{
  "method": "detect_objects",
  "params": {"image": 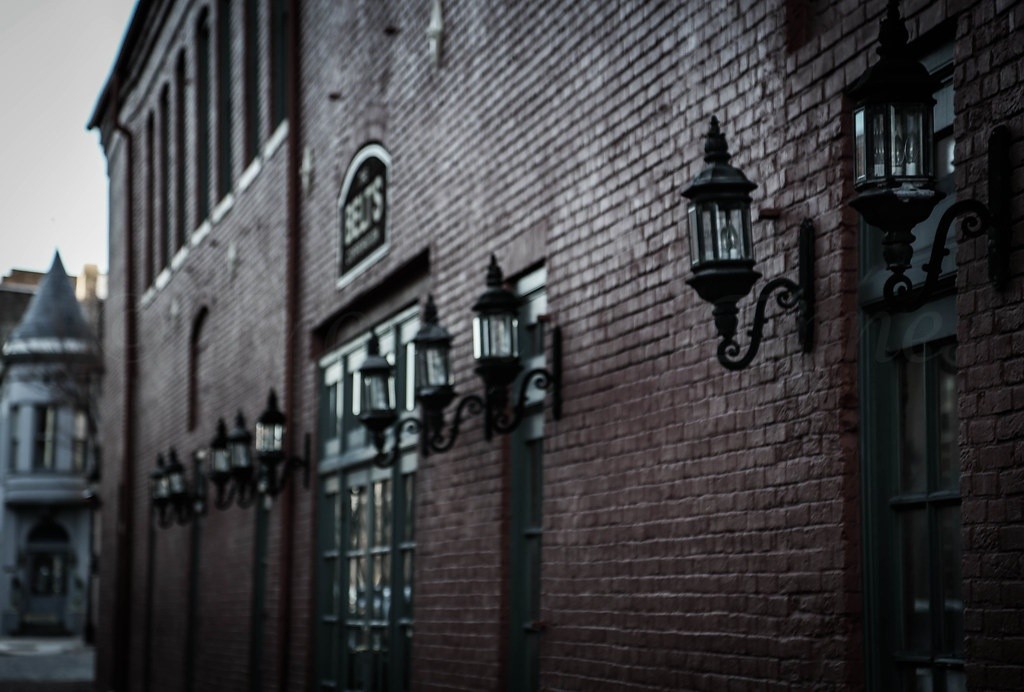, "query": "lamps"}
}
[680,117,815,372]
[150,447,172,528]
[403,293,483,458]
[357,337,423,469]
[167,446,208,527]
[208,418,233,511]
[255,387,311,498]
[470,253,562,442]
[840,1,1011,314]
[228,410,254,508]
